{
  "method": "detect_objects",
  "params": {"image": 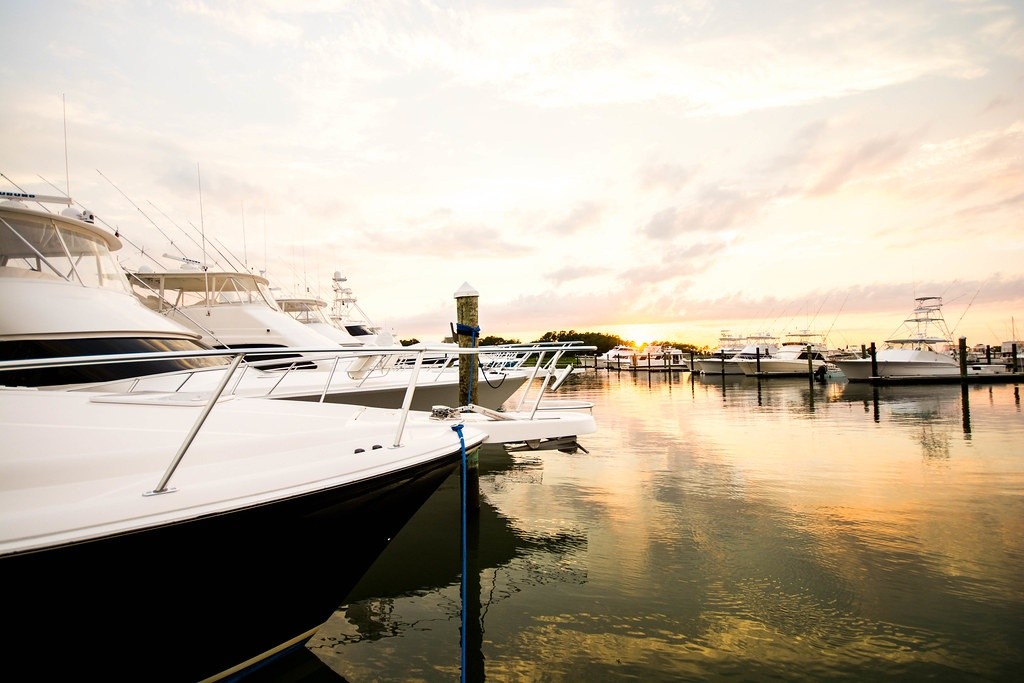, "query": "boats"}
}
[1,91,1024,449]
[0,334,603,683]
